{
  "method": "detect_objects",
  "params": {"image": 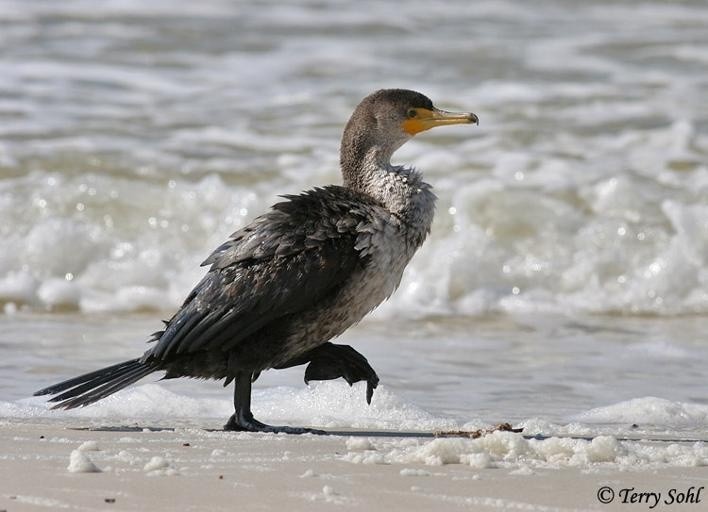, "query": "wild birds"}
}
[31,88,480,435]
